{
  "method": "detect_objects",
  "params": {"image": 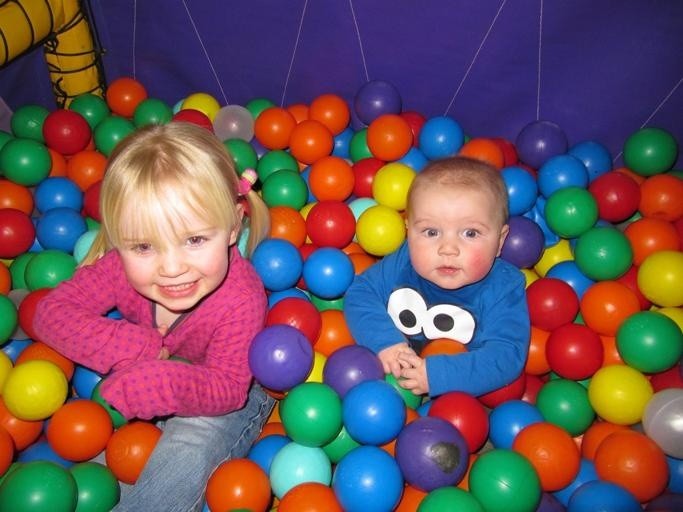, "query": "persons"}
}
[343,156,530,398]
[33,122,285,510]
